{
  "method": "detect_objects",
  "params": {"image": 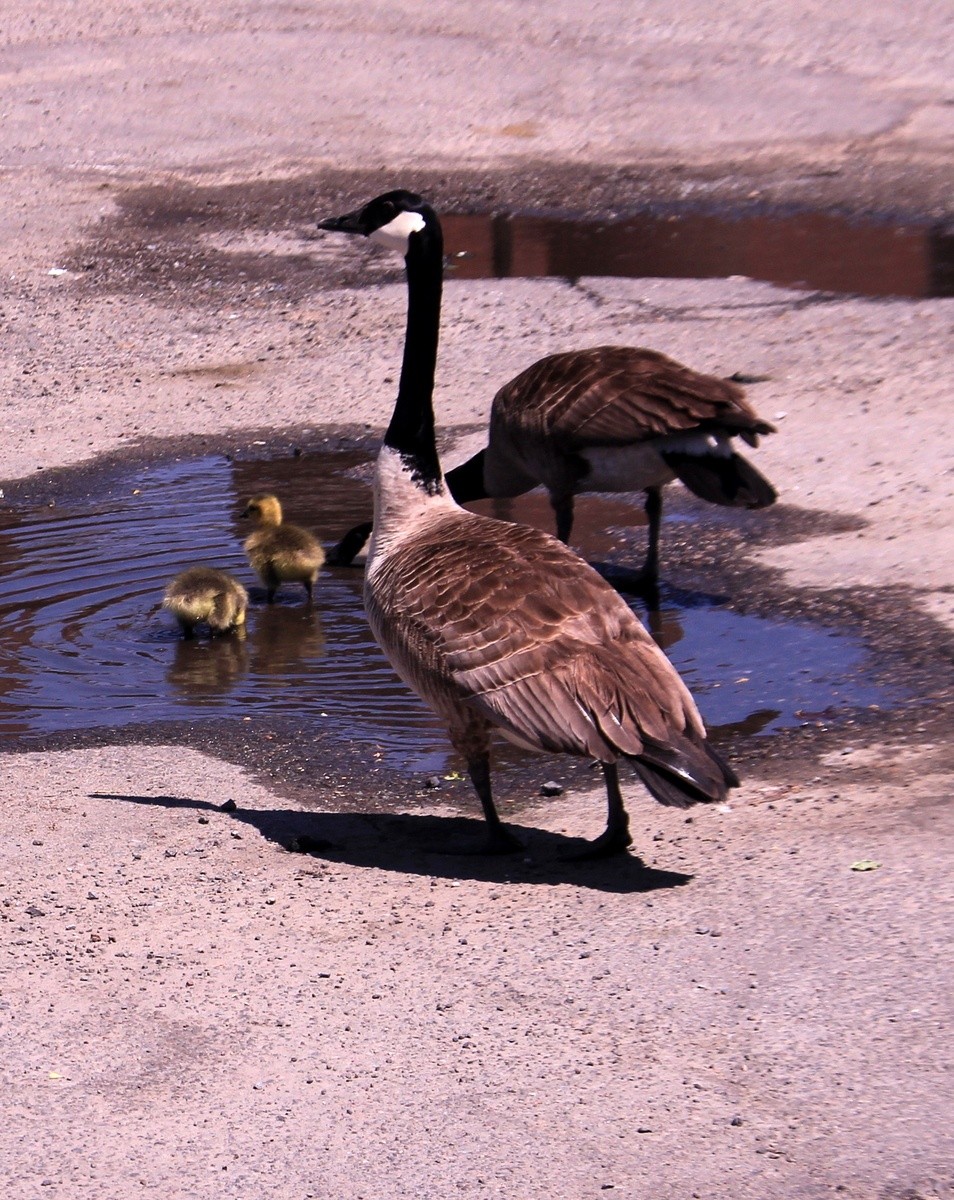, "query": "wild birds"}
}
[161,568,250,635]
[328,346,781,566]
[236,494,326,602]
[317,188,742,864]
